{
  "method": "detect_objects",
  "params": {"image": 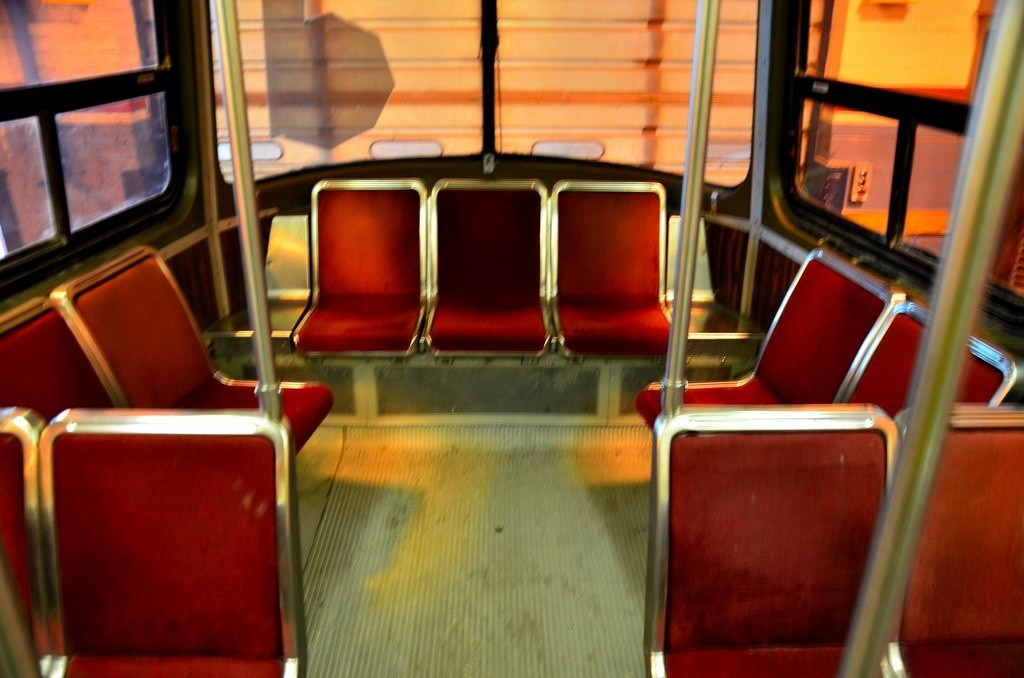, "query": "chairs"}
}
[0,180,1024,678]
